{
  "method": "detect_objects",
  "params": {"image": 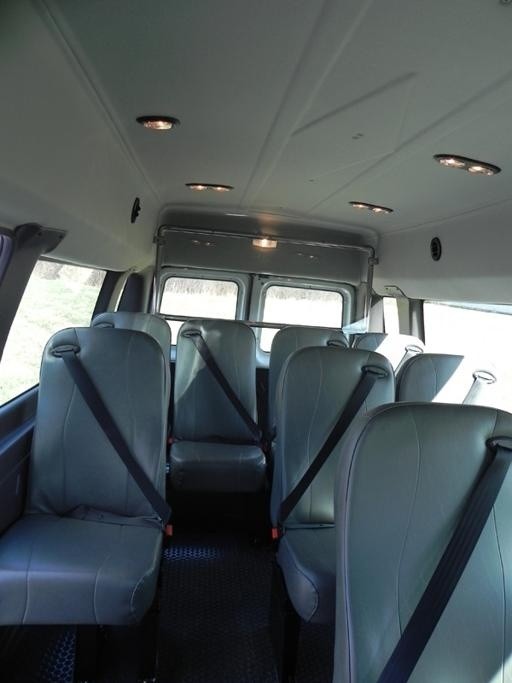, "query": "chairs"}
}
[398,352,511,412]
[333,400,512,682]
[269,346,398,627]
[1,326,170,681]
[88,311,427,490]
